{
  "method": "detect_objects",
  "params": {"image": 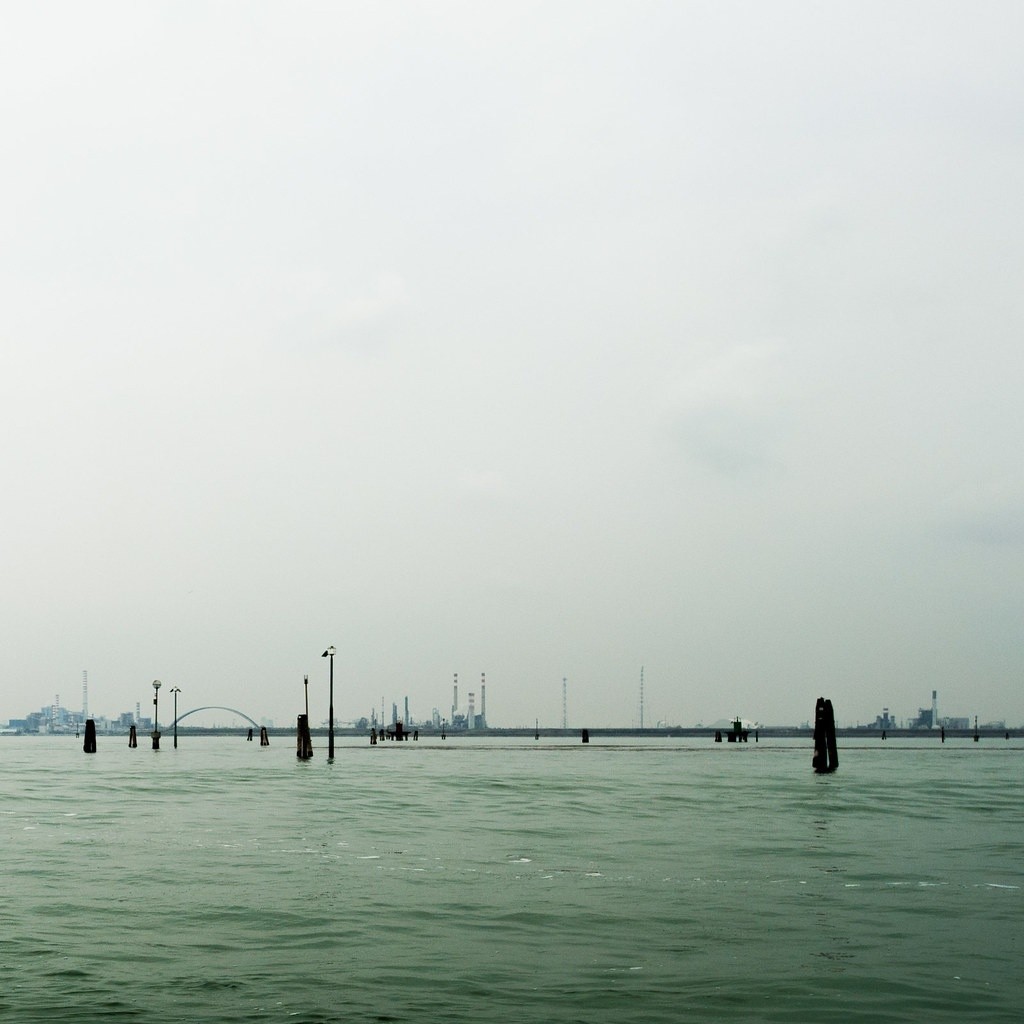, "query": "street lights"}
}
[152,679,163,751]
[170,686,180,749]
[323,646,343,765]
[304,674,309,724]
[442,718,446,740]
